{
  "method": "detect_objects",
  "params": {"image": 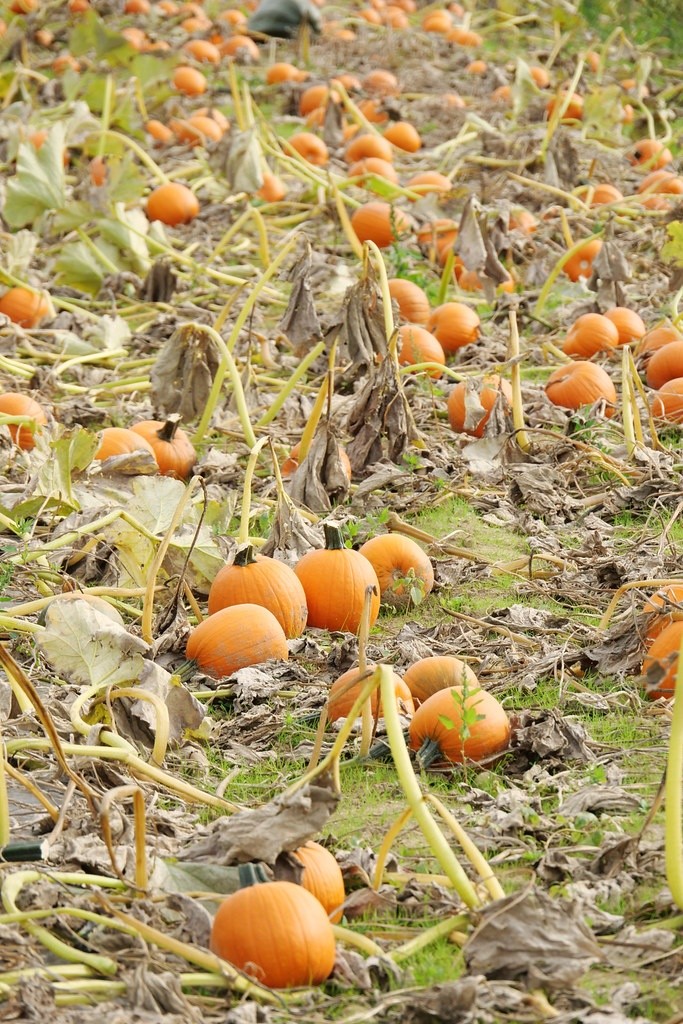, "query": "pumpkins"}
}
[209,863,337,987]
[291,841,346,924]
[0,0,683,766]
